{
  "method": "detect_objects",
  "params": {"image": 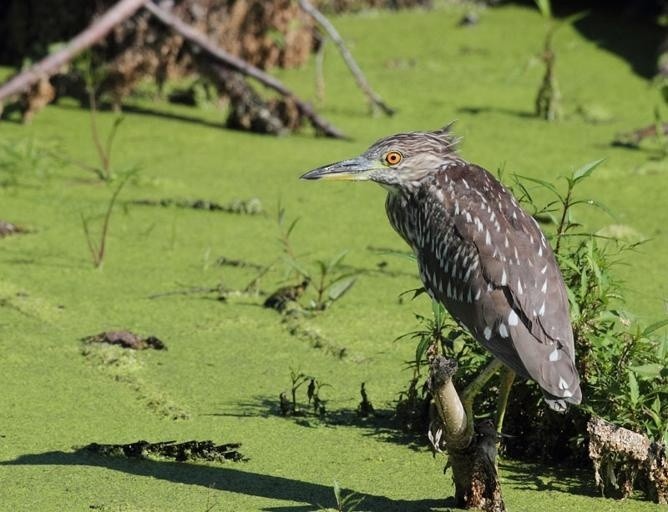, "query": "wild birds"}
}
[299,119,582,444]
